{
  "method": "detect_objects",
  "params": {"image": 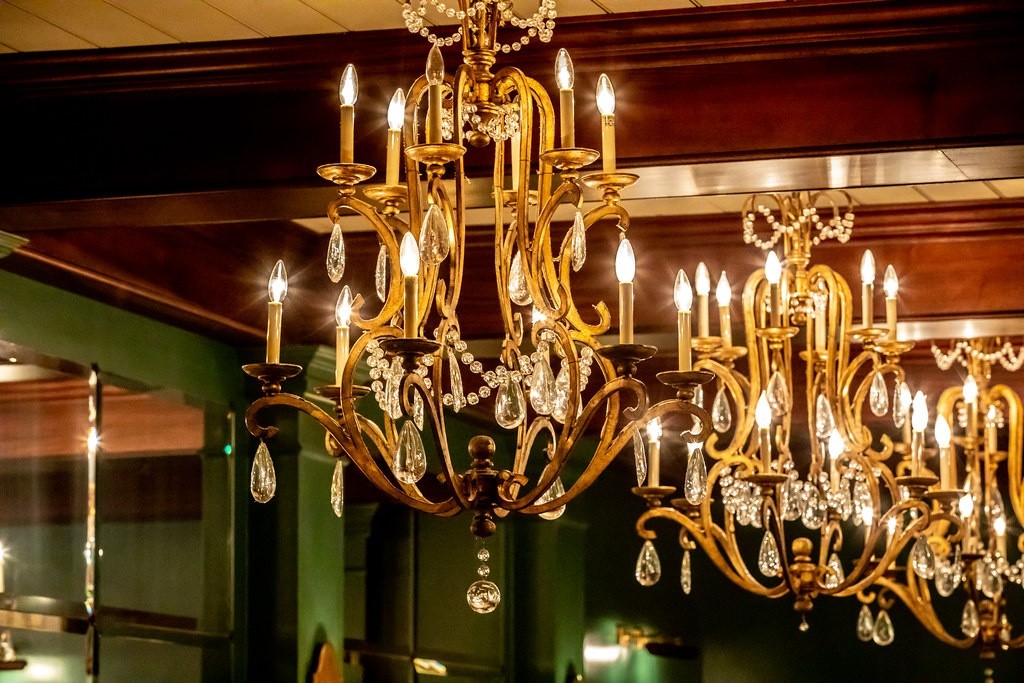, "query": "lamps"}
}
[862,336,1024,683]
[242,0,717,611]
[633,174,969,631]
